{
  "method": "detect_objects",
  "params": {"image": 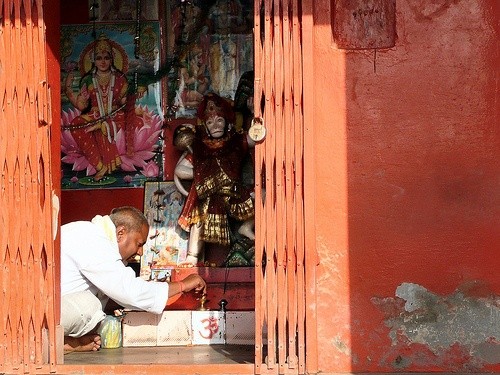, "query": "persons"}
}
[64,47,147,181]
[60,206,207,351]
[174,97,254,268]
